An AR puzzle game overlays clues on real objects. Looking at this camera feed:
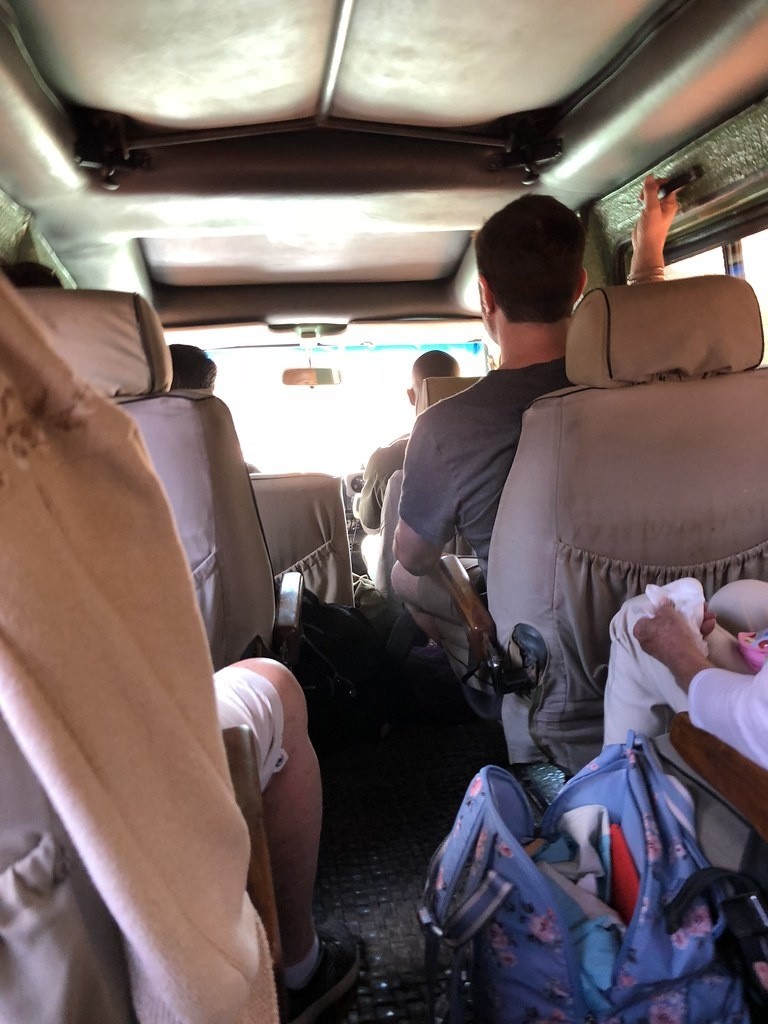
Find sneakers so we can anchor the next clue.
[274,920,360,1024]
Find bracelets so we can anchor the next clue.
[627,264,667,280]
[627,274,666,288]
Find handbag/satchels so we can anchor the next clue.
[301,588,395,736]
[417,730,768,1024]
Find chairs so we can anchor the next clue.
[433,275,768,838]
[13,286,304,684]
[647,711,768,888]
[247,472,356,608]
[361,376,484,605]
[221,722,288,1024]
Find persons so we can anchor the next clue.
[359,350,460,536]
[170,344,217,390]
[3,261,62,289]
[391,176,678,648]
[1,256,359,1024]
[601,577,768,769]
[282,369,318,385]
